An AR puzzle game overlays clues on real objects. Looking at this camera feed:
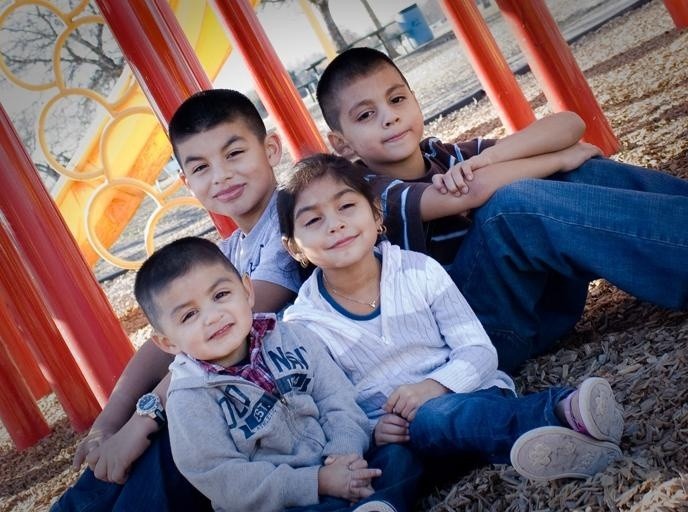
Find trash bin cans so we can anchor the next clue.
[397,3,434,46]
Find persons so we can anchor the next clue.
[277,153,626,481]
[50,90,311,511]
[134,236,419,512]
[316,47,686,375]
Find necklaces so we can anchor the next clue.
[319,273,383,310]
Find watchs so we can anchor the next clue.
[134,394,167,433]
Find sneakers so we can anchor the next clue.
[564,377,626,446]
[510,426,622,483]
